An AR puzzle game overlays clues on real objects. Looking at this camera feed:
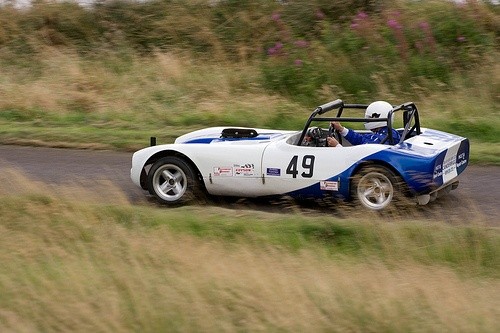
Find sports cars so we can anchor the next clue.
[130,99,470,217]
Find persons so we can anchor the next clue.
[326,101,401,148]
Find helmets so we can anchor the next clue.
[363,100,394,130]
